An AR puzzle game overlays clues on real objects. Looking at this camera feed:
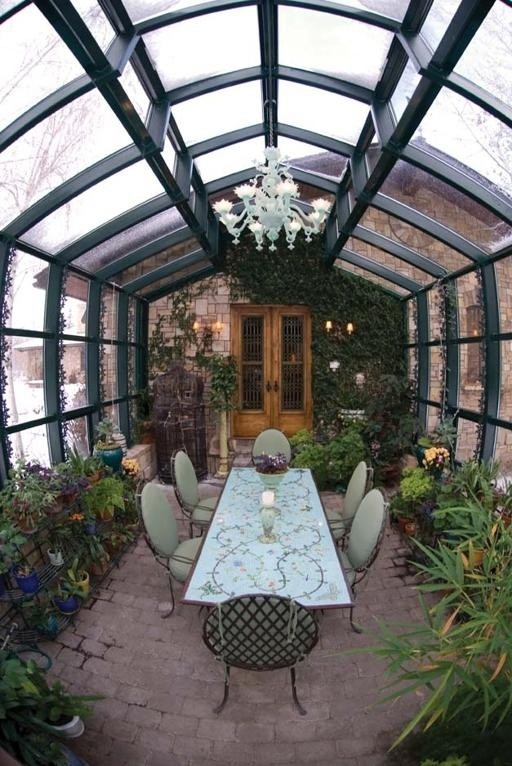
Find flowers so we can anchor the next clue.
[252,451,289,475]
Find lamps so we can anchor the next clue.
[211,98,332,253]
[192,321,223,353]
[325,321,354,372]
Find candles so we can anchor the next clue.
[262,488,274,507]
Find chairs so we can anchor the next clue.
[201,593,319,716]
[313,486,391,634]
[251,426,292,467]
[135,478,212,618]
[170,449,219,540]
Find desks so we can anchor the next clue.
[179,465,356,611]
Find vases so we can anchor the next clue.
[258,506,276,544]
[256,471,287,491]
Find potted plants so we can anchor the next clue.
[1,714,82,766]
[328,409,512,567]
[1,417,145,642]
[0,657,106,739]
